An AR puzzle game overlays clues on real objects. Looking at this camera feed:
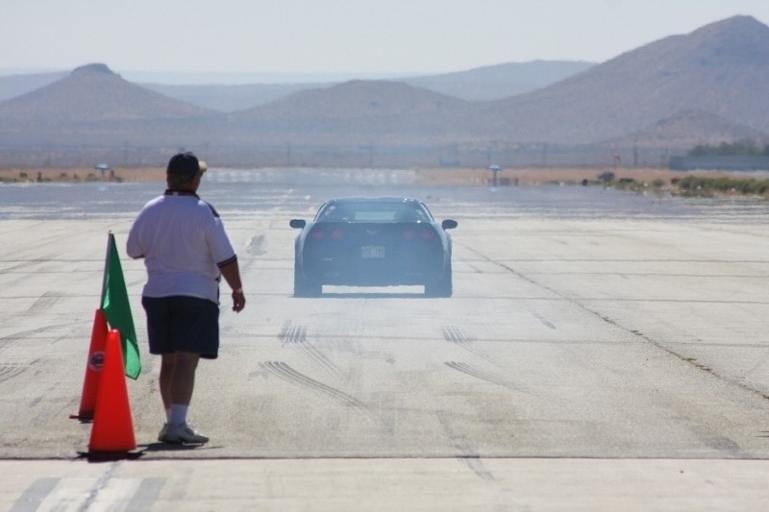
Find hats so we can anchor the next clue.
[168,154,206,176]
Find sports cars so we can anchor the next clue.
[290,196,457,298]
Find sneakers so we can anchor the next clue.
[158,423,168,441]
[164,421,208,442]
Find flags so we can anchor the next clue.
[100,233,141,380]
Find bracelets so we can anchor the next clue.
[235,288,242,294]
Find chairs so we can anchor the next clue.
[331,203,418,224]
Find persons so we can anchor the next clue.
[127,153,246,443]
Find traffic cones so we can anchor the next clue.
[78,331,147,458]
[71,308,108,420]
